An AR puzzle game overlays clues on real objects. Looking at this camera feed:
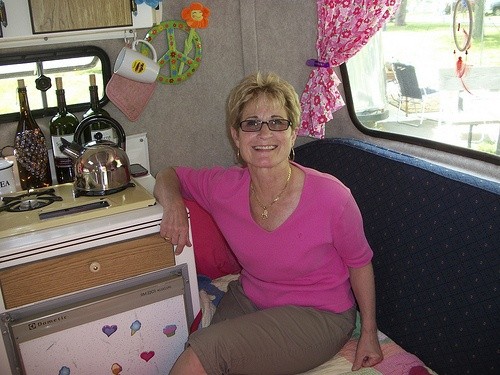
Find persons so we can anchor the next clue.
[152,70,384,375]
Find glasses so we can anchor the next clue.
[238,118,292,132]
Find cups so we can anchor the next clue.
[0,159,16,196]
[114,40,160,84]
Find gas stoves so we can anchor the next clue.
[0,175,156,240]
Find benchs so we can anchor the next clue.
[201,137,500,375]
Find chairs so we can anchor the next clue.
[381,60,441,126]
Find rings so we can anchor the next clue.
[164,236,172,240]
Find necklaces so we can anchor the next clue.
[251,166,291,217]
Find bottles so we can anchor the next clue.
[83,75,114,143]
[48,77,81,184]
[14,79,53,190]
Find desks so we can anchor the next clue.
[419,114,500,156]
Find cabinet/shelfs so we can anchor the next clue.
[0,231,201,375]
[28,0,134,34]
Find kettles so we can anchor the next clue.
[61,114,132,197]
